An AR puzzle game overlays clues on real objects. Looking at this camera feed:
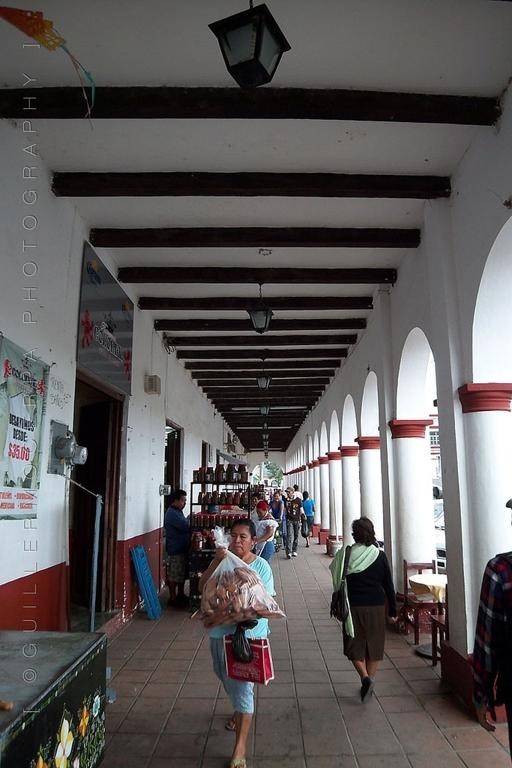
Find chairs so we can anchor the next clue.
[400,558,450,666]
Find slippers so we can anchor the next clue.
[225,713,237,731]
[230,757,247,767]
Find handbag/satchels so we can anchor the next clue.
[223,633,274,686]
[330,582,348,622]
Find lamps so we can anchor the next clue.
[247,282,274,458]
[207,1,292,91]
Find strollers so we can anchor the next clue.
[272,516,284,552]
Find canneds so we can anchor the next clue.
[191,463,249,552]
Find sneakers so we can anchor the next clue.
[287,551,297,558]
[168,594,189,608]
[360,678,374,703]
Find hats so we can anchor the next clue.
[257,501,267,508]
[285,486,295,492]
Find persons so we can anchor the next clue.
[196,518,277,768]
[164,489,195,609]
[331,516,400,703]
[249,484,316,563]
[471,552,511,760]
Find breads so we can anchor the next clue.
[200,567,286,627]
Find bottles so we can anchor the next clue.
[198,462,248,484]
[198,491,249,506]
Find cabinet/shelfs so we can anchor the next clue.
[188,480,249,600]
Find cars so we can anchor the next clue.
[433,481,447,576]
